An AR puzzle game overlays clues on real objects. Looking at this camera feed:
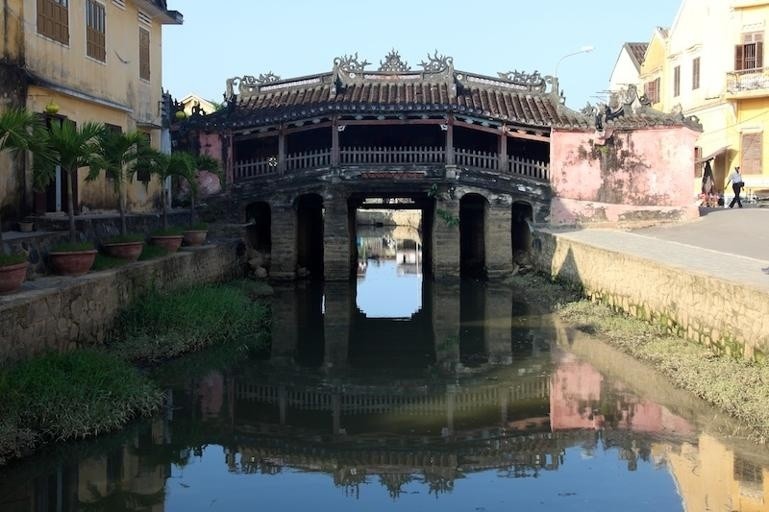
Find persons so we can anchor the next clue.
[723,165,746,208]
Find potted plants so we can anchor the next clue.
[125,149,229,255]
[1,107,148,295]
